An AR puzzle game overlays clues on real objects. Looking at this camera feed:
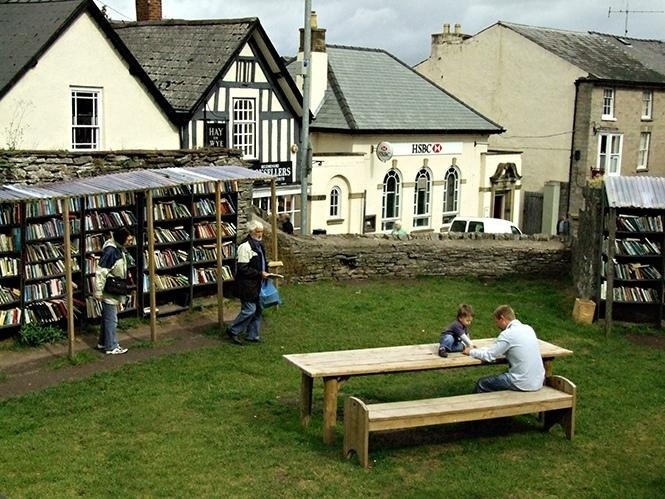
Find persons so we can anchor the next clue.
[389,220,407,235]
[556,214,567,236]
[280,214,295,235]
[93,227,134,356]
[227,220,270,343]
[438,303,479,359]
[462,304,548,393]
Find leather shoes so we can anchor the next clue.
[228,332,241,343]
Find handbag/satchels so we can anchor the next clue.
[105,276,127,295]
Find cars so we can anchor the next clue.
[449,215,526,235]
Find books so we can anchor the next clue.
[0,180,239,324]
[601,214,664,300]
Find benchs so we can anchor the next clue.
[343,375,577,468]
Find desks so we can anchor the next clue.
[282,338,573,444]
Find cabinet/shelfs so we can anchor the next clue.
[1,165,279,356]
[596,175,665,337]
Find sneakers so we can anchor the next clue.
[440,352,448,356]
[98,344,105,348]
[106,344,128,354]
